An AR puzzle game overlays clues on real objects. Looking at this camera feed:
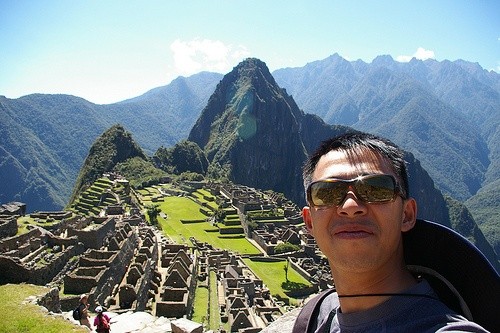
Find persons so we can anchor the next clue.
[79,294,91,331]
[259,133,493,333]
[94,306,111,333]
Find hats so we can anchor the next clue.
[403,218,500,333]
[79,294,89,301]
[95,306,103,312]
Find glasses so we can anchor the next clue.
[306,174,407,211]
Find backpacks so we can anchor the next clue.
[96,315,111,333]
[73,304,86,320]
